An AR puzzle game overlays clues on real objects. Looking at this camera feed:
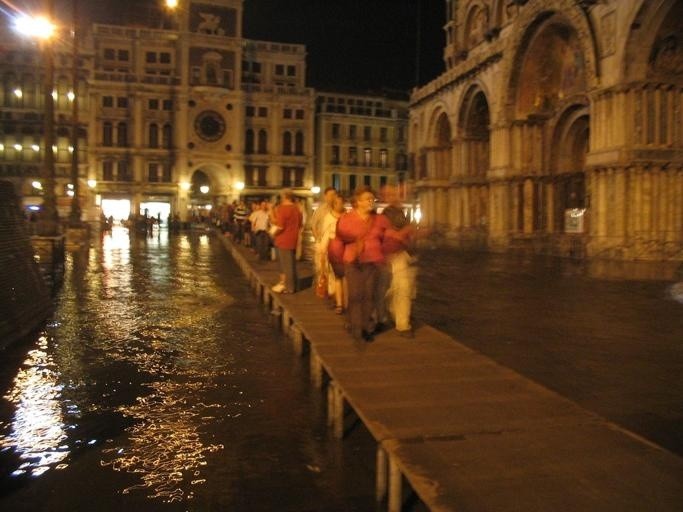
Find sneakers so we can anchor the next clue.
[273,281,296,293]
[393,329,415,338]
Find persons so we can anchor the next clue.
[190,183,423,348]
